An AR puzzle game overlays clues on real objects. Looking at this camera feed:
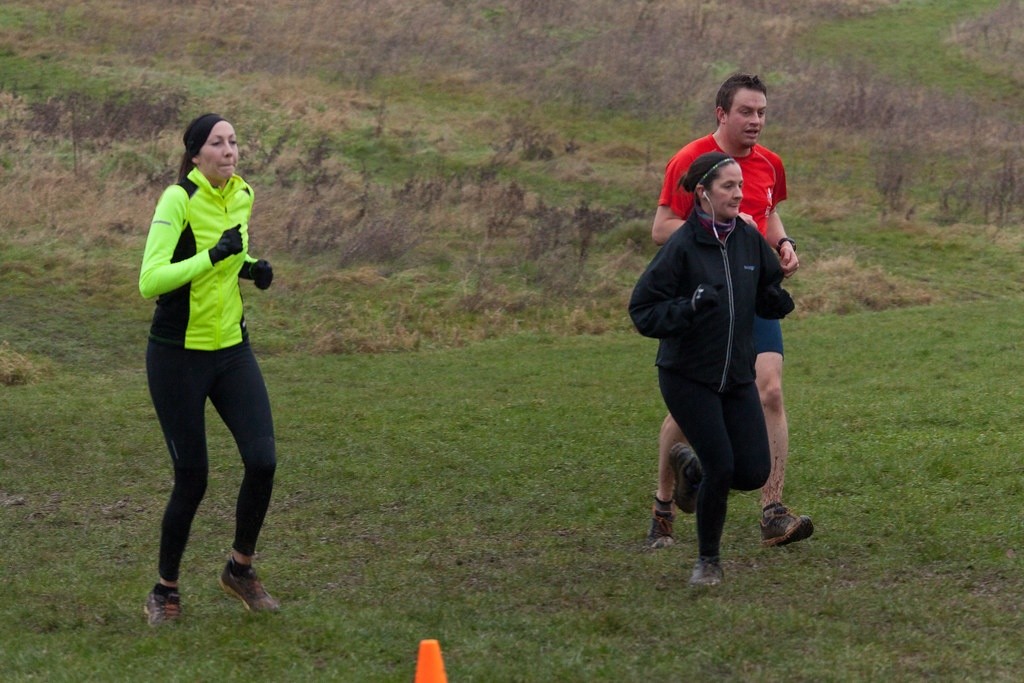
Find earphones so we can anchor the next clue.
[703,192,711,203]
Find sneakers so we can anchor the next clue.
[689,558,722,587]
[646,506,673,539]
[669,442,697,515]
[218,561,279,616]
[143,593,180,628]
[760,509,813,548]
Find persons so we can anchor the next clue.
[650,74,813,548]
[628,153,796,586]
[140,113,279,618]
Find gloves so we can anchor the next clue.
[692,284,719,318]
[208,224,244,265]
[778,289,796,316]
[252,258,272,290]
